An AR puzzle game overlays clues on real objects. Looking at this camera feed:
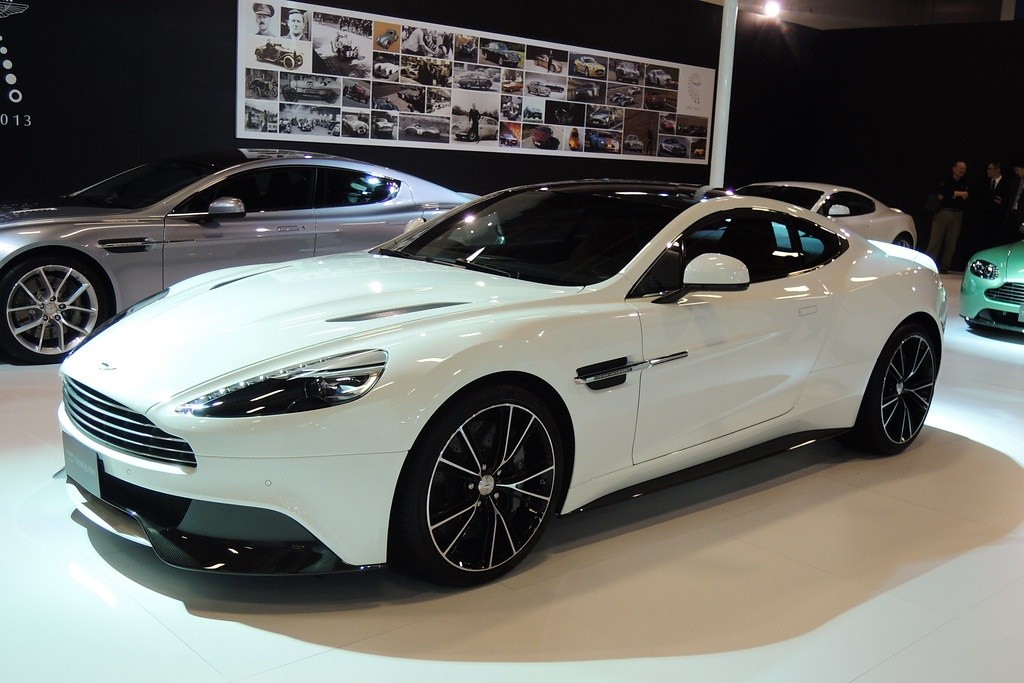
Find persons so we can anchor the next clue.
[547,50,553,73]
[645,124,654,156]
[457,34,476,54]
[926,162,969,273]
[339,16,372,37]
[286,10,308,41]
[253,3,275,36]
[469,102,482,143]
[247,109,278,132]
[401,25,453,59]
[983,159,1024,249]
[508,102,518,120]
[400,57,452,85]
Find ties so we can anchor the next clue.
[992,180,995,189]
[1011,180,1024,211]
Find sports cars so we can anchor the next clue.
[405,123,441,138]
[59,181,932,585]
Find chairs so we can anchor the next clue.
[711,216,779,279]
[544,198,641,264]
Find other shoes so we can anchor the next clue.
[940,268,947,274]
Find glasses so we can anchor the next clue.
[987,168,997,170]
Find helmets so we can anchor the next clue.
[355,82,357,85]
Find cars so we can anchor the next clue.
[501,79,564,149]
[590,131,620,152]
[647,91,667,106]
[0,148,507,372]
[958,240,1024,334]
[343,114,369,137]
[376,29,399,49]
[253,41,303,70]
[458,71,492,90]
[647,69,671,87]
[589,108,617,129]
[613,86,642,107]
[660,118,707,159]
[616,62,641,85]
[481,41,521,66]
[625,134,644,152]
[690,183,918,258]
[373,117,394,134]
[535,54,564,72]
[574,56,606,78]
[569,128,581,151]
[452,116,498,141]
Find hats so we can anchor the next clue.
[252,2,275,17]
